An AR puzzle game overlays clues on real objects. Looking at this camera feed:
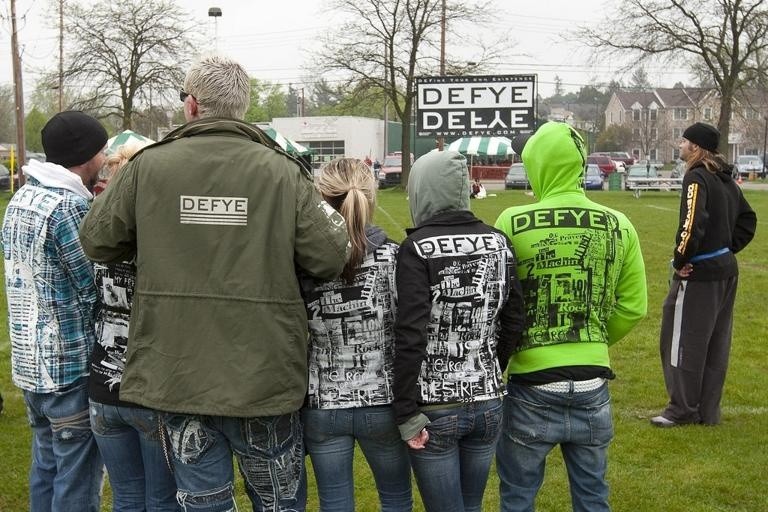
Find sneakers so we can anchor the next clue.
[649,414,678,427]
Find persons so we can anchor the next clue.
[731,162,740,182]
[493,122,647,512]
[80,58,351,512]
[87,147,181,511]
[1,110,104,512]
[651,123,757,426]
[295,159,412,512]
[394,150,525,512]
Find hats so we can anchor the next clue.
[683,121,722,155]
[40,110,109,168]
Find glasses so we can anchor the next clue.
[179,88,196,104]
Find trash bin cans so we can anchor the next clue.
[608,173,621,191]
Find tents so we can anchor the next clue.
[106,129,157,155]
[451,136,517,179]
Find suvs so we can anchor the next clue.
[377,151,414,189]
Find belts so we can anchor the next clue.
[528,375,608,395]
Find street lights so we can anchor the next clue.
[166,108,174,132]
[561,100,570,119]
[208,7,222,57]
[631,72,649,155]
[589,85,598,153]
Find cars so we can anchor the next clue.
[0,162,30,192]
[503,162,532,189]
[580,151,768,191]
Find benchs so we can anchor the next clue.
[625,176,682,198]
